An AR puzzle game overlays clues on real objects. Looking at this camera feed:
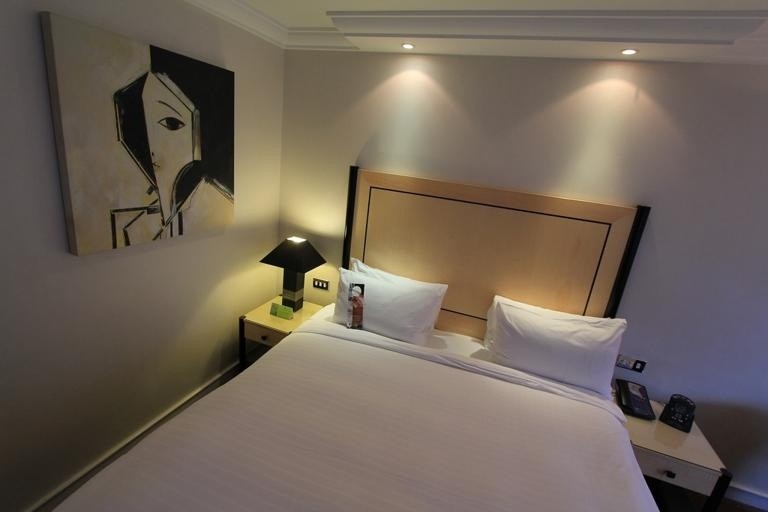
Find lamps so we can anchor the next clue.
[259,235,327,312]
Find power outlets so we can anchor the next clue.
[615,353,647,374]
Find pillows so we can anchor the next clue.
[347,258,439,285]
[333,268,451,346]
[484,296,629,399]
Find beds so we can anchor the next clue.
[59,162,661,512]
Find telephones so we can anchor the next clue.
[615,379,655,420]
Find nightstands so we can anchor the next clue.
[613,390,733,512]
[241,295,324,373]
